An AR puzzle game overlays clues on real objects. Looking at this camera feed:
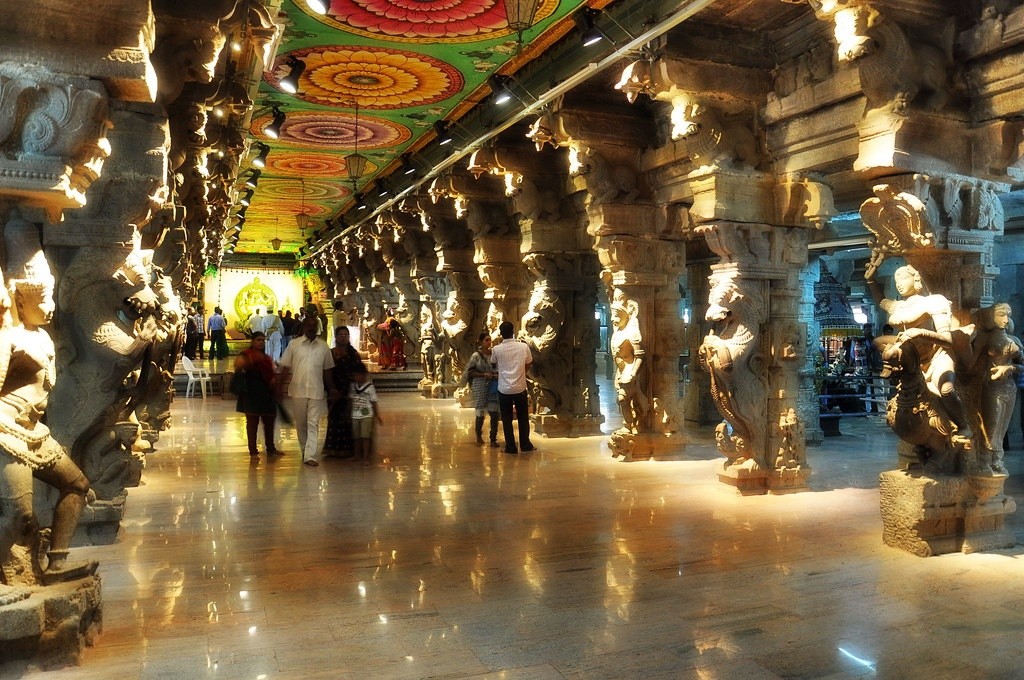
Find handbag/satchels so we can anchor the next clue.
[492,379,500,393]
[229,351,251,393]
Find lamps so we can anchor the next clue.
[433,118,476,145]
[306,0,330,14]
[271,221,282,250]
[227,168,261,253]
[251,141,271,167]
[488,73,538,107]
[573,5,656,64]
[374,178,388,197]
[302,220,335,260]
[400,152,433,174]
[279,57,306,94]
[354,193,367,210]
[503,0,539,45]
[265,105,287,138]
[342,104,368,190]
[296,181,311,236]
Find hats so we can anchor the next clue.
[864,324,872,329]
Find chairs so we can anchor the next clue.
[182,355,212,399]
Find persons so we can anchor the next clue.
[185,306,206,360]
[485,312,501,350]
[883,264,1024,474]
[863,323,893,384]
[0,278,97,578]
[611,304,649,434]
[207,309,228,360]
[491,322,537,453]
[377,311,408,369]
[418,311,439,383]
[467,333,501,447]
[247,305,324,366]
[236,317,384,466]
